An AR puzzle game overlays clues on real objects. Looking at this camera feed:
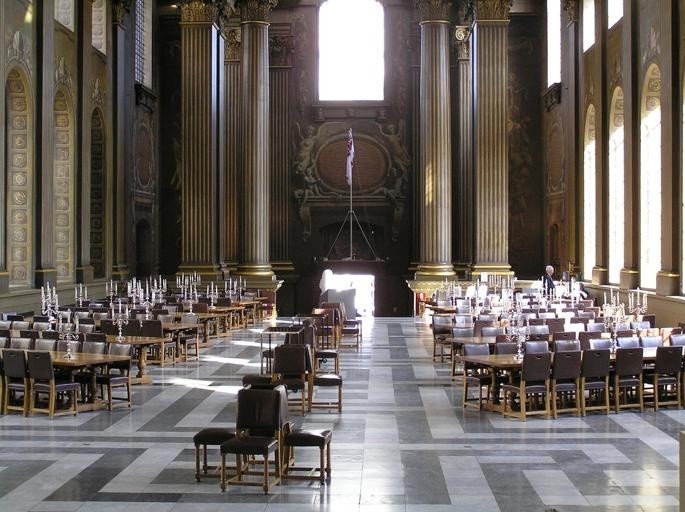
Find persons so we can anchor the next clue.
[370,170,403,212]
[292,119,319,174]
[541,263,559,294]
[375,119,413,170]
[299,167,321,209]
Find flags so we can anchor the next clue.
[344,126,358,189]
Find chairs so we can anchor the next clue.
[416,285,685,424]
